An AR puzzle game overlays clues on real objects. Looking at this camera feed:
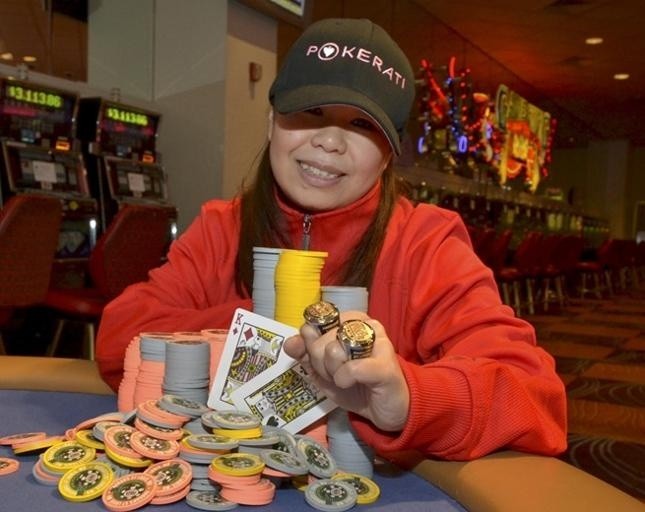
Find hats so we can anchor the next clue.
[268,17,416,158]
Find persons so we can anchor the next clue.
[94,17,568,472]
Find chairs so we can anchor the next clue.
[0,191,181,360]
[471,227,645,319]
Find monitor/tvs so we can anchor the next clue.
[2,138,91,199]
[100,155,170,204]
[237,0,314,32]
[43,0,89,25]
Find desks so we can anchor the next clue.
[1,353,645,512]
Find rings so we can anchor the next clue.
[303,300,339,335]
[336,319,375,360]
